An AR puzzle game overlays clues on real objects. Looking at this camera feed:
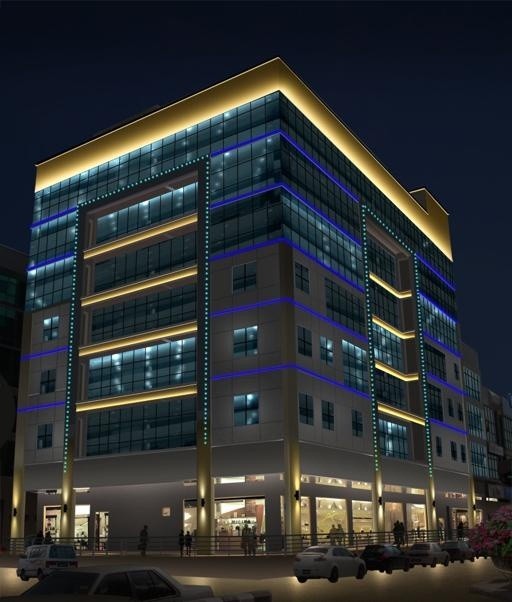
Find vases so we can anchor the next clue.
[489,556,512,570]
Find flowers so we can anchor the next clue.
[471,502,512,574]
